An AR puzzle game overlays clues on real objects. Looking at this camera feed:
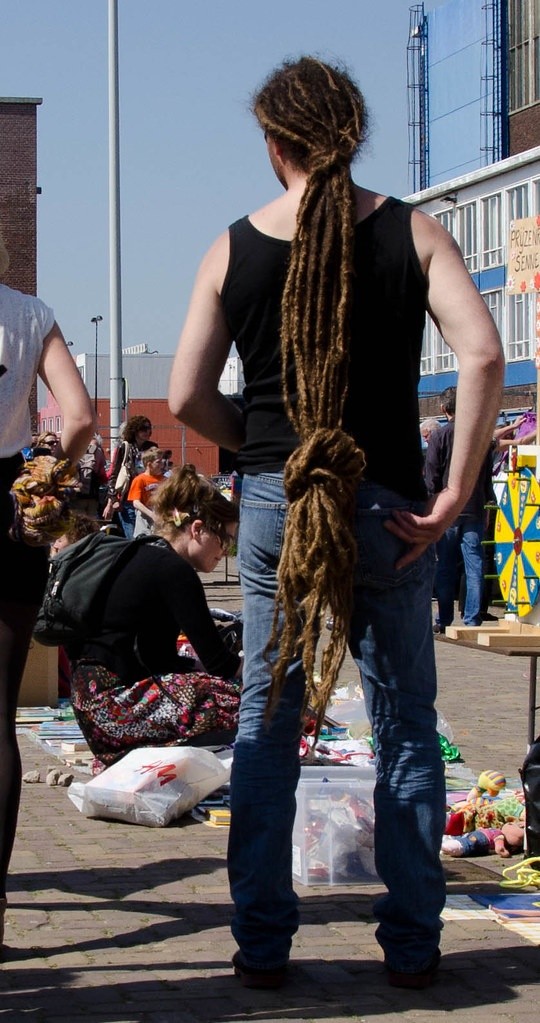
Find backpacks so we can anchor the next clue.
[34,528,173,647]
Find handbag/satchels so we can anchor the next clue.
[115,441,130,494]
[81,747,232,828]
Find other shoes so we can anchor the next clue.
[480,612,498,621]
[431,623,445,634]
[231,950,287,988]
[386,946,440,988]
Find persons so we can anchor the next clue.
[0,241,98,954]
[22,415,242,766]
[420,387,537,634]
[169,57,506,988]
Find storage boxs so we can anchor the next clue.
[291,766,385,886]
[17,634,60,707]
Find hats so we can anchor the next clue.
[142,446,173,466]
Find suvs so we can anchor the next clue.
[209,476,232,489]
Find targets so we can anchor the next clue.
[492,466,539,619]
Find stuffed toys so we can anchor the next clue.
[441,771,525,857]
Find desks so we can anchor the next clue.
[438,618,540,859]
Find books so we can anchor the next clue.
[203,806,231,826]
[15,707,89,751]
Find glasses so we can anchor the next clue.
[42,440,59,446]
[185,517,236,551]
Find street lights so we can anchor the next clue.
[90,316,103,413]
